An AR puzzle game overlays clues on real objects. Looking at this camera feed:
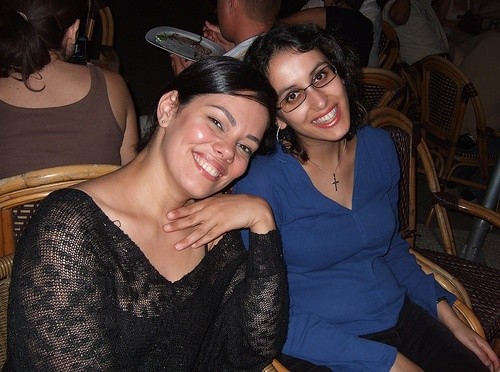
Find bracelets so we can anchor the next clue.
[434,296,447,304]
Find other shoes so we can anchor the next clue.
[460,190,478,204]
[444,187,460,199]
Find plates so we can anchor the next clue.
[145,25,226,63]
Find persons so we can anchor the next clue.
[2,56,289,372]
[233,24,500,372]
[0,0,139,180]
[169,0,456,87]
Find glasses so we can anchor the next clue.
[277,60,338,112]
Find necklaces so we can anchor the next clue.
[309,143,343,191]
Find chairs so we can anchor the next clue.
[0,0,500,372]
